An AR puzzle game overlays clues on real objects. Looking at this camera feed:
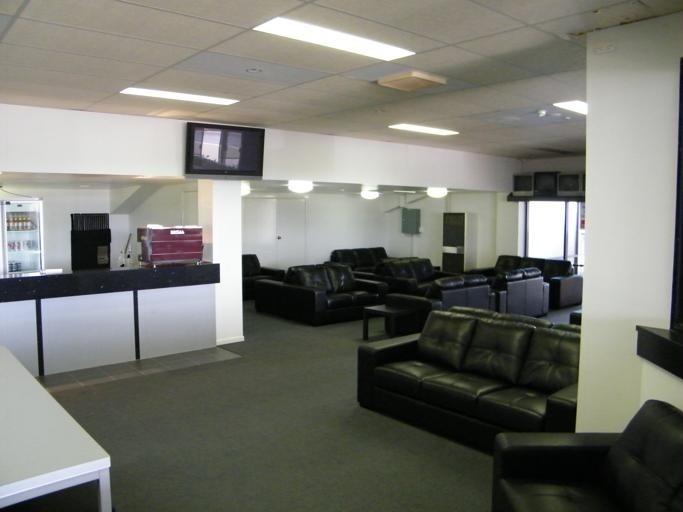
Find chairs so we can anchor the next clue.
[492,399,683,511]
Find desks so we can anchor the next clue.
[1,346,112,512]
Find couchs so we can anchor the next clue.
[255,263,390,328]
[463,254,582,310]
[361,266,552,344]
[323,248,420,281]
[243,253,287,305]
[385,256,462,296]
[353,304,583,457]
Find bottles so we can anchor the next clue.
[7,215,32,231]
[7,240,37,252]
[7,259,22,272]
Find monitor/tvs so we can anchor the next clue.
[185,121,265,178]
[511,172,534,197]
[558,172,584,197]
[534,171,558,197]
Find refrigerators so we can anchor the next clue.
[0,198,43,274]
